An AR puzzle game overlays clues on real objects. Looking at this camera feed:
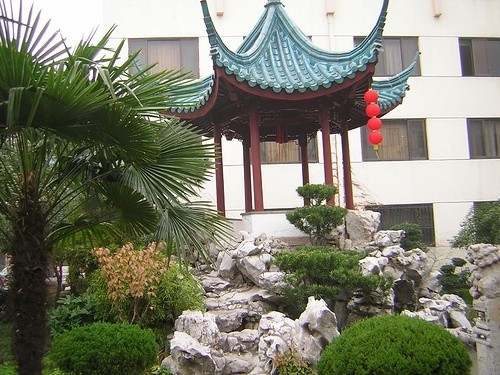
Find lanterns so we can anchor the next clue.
[364,87,383,159]
